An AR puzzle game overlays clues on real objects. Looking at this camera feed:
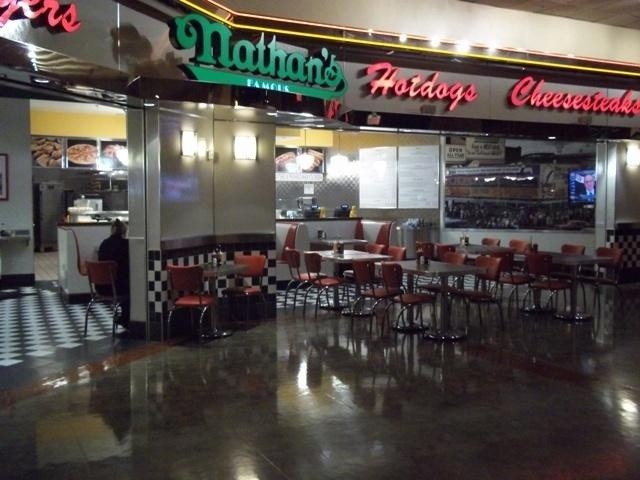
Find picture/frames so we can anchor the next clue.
[0,153,10,201]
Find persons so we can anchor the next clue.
[95,217,130,329]
[93,337,136,443]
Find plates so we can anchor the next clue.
[66,144,97,165]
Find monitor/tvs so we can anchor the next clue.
[568,167,596,204]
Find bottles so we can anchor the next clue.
[333,239,344,255]
[416,245,425,265]
[213,245,224,267]
[349,205,359,217]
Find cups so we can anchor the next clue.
[320,207,326,218]
